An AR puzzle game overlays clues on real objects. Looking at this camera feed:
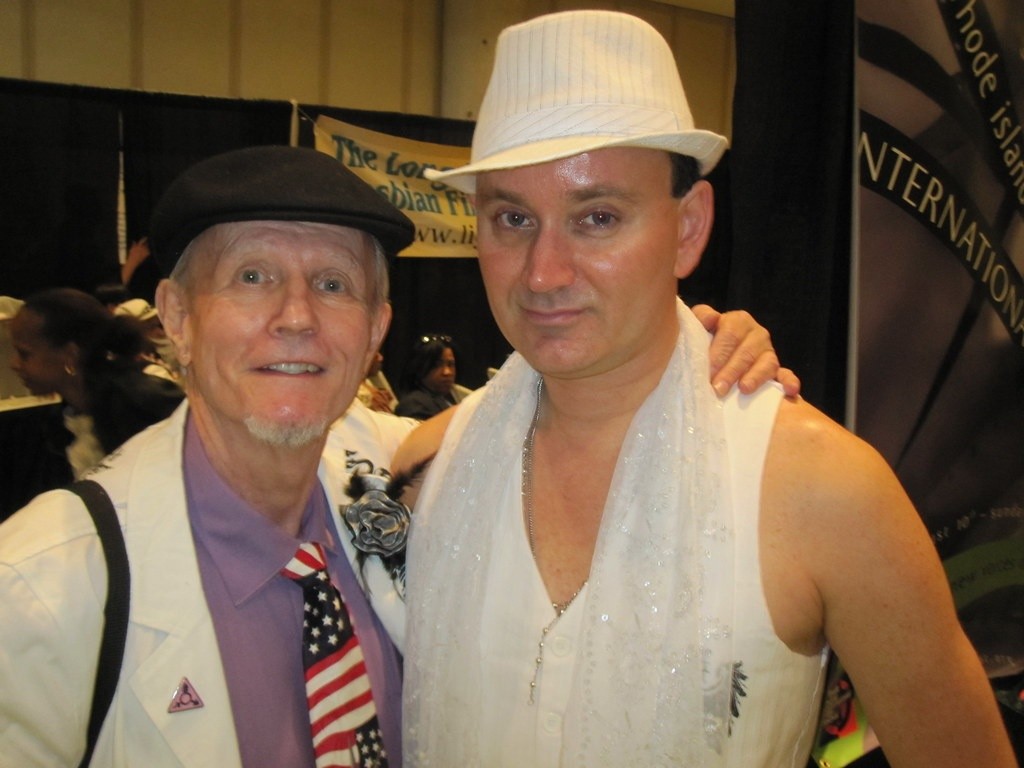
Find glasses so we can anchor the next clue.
[421,335,453,343]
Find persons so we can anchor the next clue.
[0,145,799,768]
[356,352,398,414]
[390,10,1019,768]
[394,332,474,422]
[7,236,186,513]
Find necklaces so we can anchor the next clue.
[521,377,586,705]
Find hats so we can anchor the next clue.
[147,144,415,278]
[422,9,729,194]
